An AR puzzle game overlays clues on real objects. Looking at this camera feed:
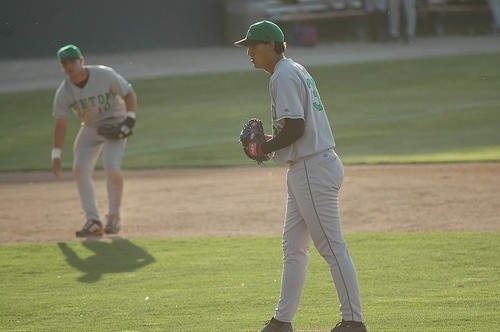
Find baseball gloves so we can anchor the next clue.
[239,117,274,163]
[97,125,134,141]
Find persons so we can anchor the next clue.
[52,45,137,236]
[234,20,367,332]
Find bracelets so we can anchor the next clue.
[52,149,61,158]
[127,112,136,119]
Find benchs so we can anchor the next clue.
[415,5,492,14]
[268,8,362,22]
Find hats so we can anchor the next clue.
[57,44,82,63]
[235,20,284,46]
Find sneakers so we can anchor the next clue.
[76,219,102,237]
[331,322,366,332]
[105,218,121,233]
[261,317,293,332]
